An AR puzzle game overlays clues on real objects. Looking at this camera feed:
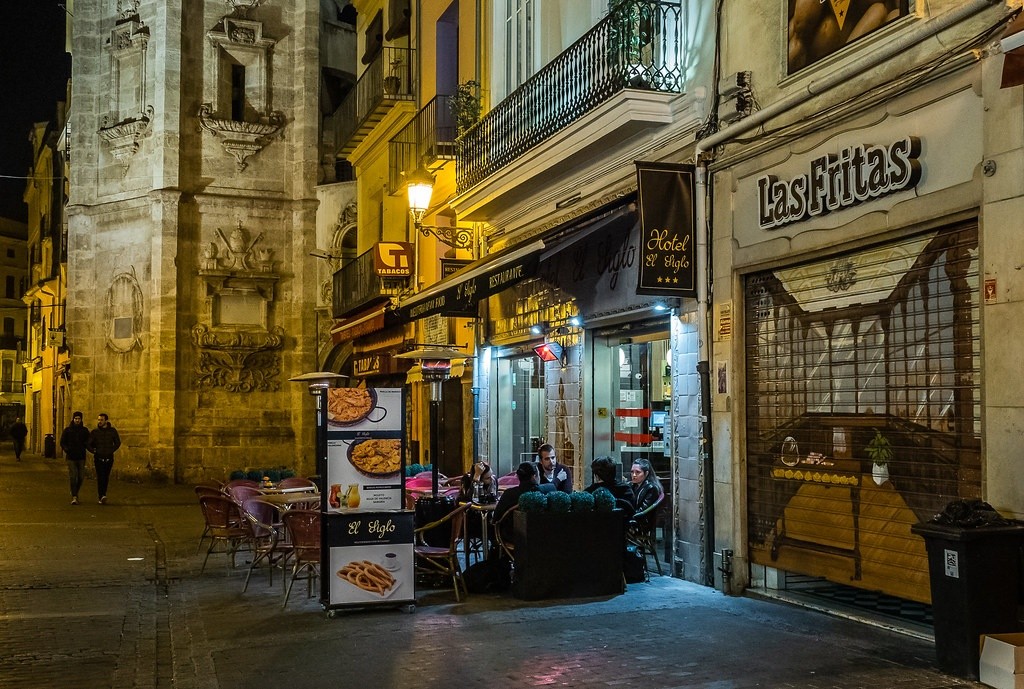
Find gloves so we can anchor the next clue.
[557,468,567,481]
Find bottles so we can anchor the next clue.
[258,481,273,488]
[473,482,484,499]
[329,483,361,509]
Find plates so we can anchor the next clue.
[380,560,401,572]
[335,563,402,599]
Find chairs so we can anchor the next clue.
[613,481,665,580]
[194,476,322,607]
[412,501,472,604]
[490,504,519,592]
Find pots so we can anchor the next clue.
[342,439,401,478]
[327,389,387,427]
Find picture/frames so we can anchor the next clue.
[775,1,925,89]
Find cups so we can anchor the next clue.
[383,552,399,568]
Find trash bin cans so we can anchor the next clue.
[44,434,56,458]
[911,520,1024,681]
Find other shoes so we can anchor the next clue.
[98,496,107,504]
[70,497,79,504]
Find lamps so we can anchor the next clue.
[403,160,472,251]
[383,52,404,96]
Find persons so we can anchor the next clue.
[60,412,90,505]
[487,461,557,584]
[457,461,499,538]
[627,458,660,534]
[10,417,28,462]
[536,444,572,495]
[86,413,121,504]
[583,456,636,523]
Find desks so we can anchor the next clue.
[254,486,315,536]
[256,489,322,583]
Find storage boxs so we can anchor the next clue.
[979,634,1024,689]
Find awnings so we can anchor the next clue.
[331,300,390,346]
[400,199,621,324]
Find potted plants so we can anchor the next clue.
[513,486,626,602]
[447,79,486,159]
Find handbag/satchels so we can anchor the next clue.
[94,455,114,465]
